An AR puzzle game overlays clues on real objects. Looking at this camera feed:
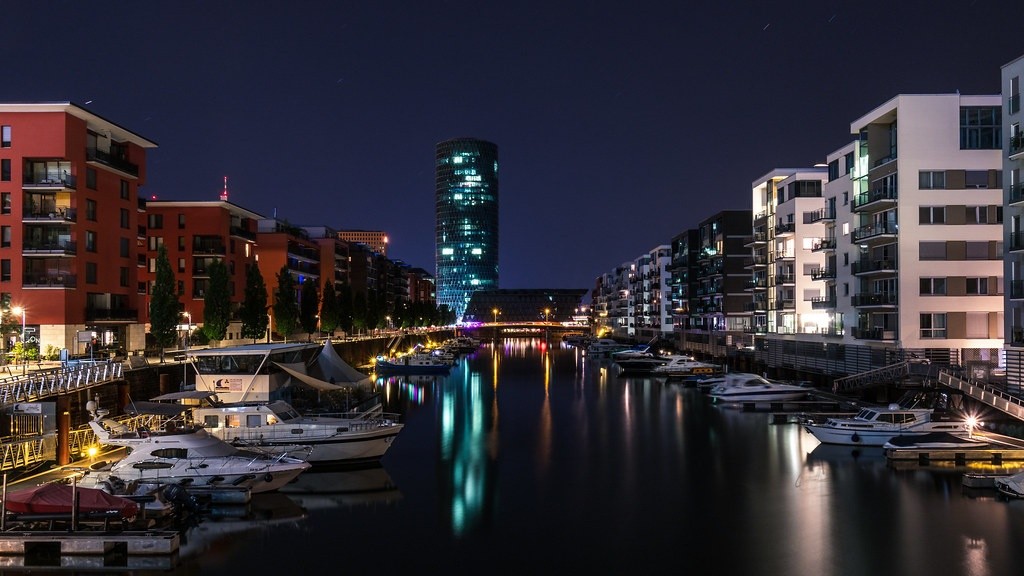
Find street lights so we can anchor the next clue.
[182,311,192,346]
[492,308,499,322]
[545,308,550,321]
[10,304,27,349]
[315,315,320,337]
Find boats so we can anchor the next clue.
[67,393,314,495]
[0,473,180,555]
[563,334,634,353]
[798,390,969,447]
[149,391,404,469]
[375,336,480,375]
[612,335,815,403]
[38,448,201,531]
[883,432,991,451]
[1,481,137,513]
